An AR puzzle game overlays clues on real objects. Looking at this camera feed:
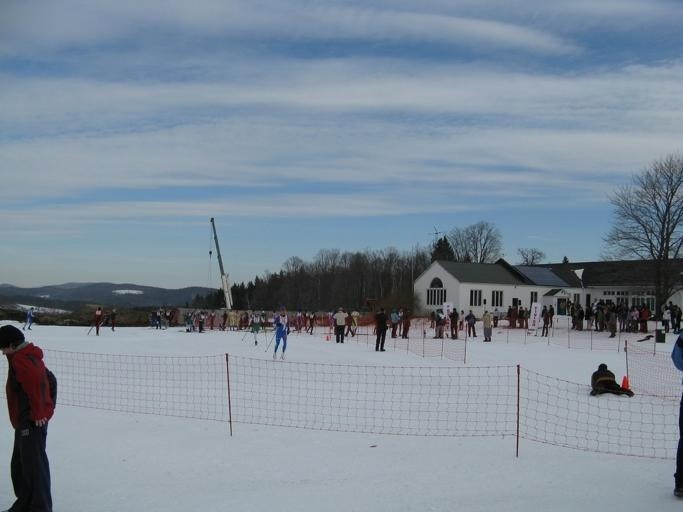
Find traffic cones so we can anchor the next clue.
[325,336,329,341]
[620,376,627,388]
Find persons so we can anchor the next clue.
[506,304,533,329]
[95,307,102,336]
[374,306,410,352]
[155,306,173,330]
[220,309,266,334]
[493,307,499,328]
[483,310,492,342]
[590,363,635,397]
[23,307,35,331]
[0,325,57,512]
[185,309,216,333]
[671,331,683,497]
[541,304,554,337]
[111,308,117,331]
[559,299,651,338]
[273,306,289,360]
[661,300,682,334]
[332,306,359,343]
[429,305,478,339]
[293,307,317,335]
